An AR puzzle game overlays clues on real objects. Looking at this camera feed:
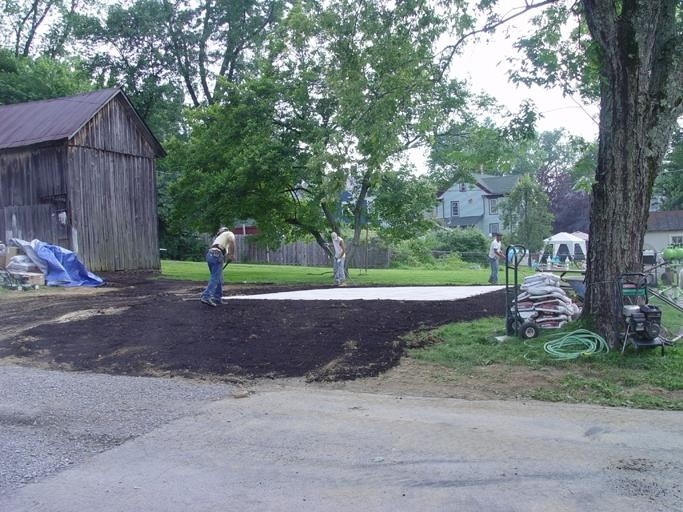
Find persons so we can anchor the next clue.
[488,232,506,284]
[323,232,346,285]
[199,226,236,307]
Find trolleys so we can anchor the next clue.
[501,243,556,338]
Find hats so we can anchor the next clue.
[217,227,228,235]
[331,232,337,240]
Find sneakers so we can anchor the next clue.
[201,297,228,307]
